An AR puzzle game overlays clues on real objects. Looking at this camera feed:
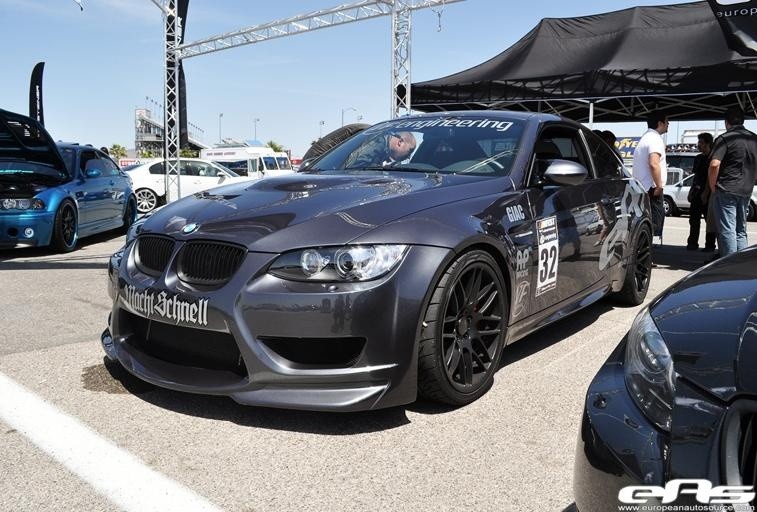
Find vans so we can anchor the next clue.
[201,147,295,186]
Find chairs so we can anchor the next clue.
[535,141,562,157]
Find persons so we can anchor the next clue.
[686,132,717,252]
[301,124,416,168]
[703,105,757,264]
[634,110,669,268]
[594,128,624,161]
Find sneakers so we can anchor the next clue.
[703,256,721,264]
[686,244,714,251]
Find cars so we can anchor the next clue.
[100,110,654,414]
[573,237,756,509]
[663,170,756,220]
[0,111,139,254]
[121,158,248,213]
